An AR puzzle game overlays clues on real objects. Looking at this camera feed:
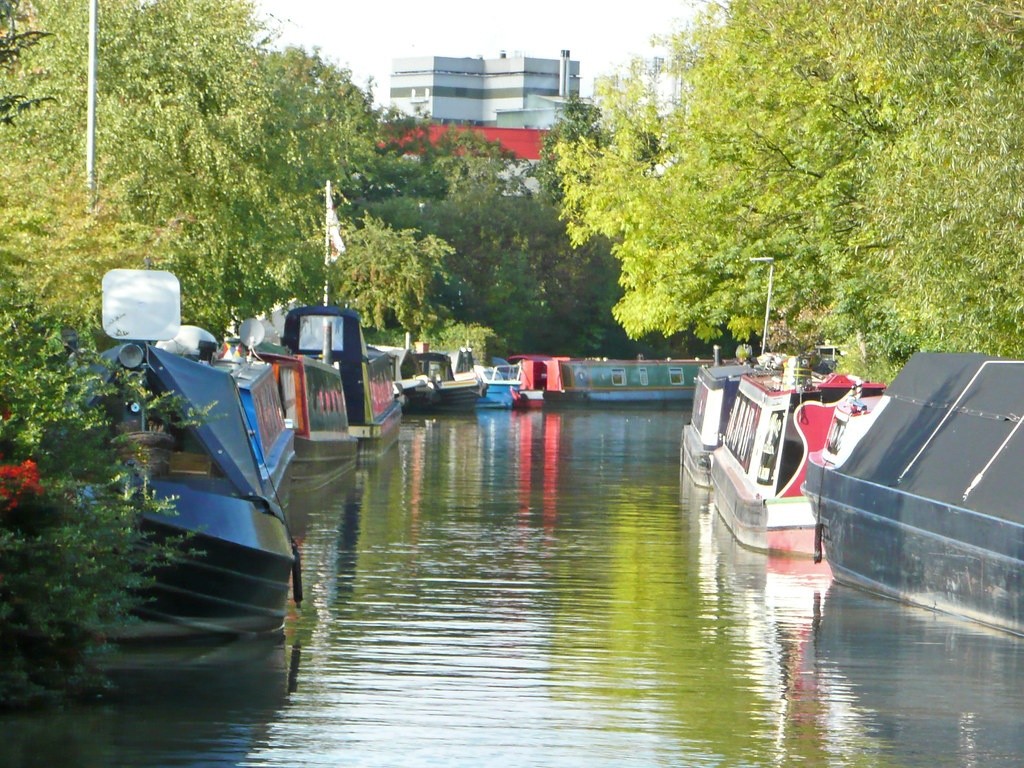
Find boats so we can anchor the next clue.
[678,256,888,557]
[0,180,523,639]
[802,354,1024,638]
[508,356,758,402]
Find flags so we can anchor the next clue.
[326,193,346,263]
